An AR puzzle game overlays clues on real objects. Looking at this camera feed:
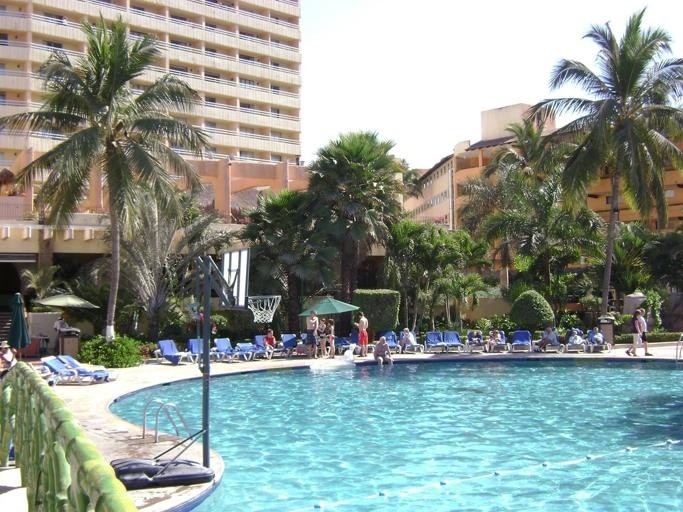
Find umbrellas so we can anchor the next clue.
[7,292,31,360]
[35,291,100,324]
[298,296,360,317]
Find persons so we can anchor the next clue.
[318,318,328,358]
[261,327,276,358]
[533,326,556,352]
[304,310,319,360]
[353,311,369,357]
[569,330,586,344]
[631,308,653,356]
[0,339,17,369]
[488,330,500,345]
[471,330,482,343]
[373,335,394,366]
[326,318,335,360]
[587,327,605,345]
[625,309,643,356]
[399,328,415,346]
[52,316,65,355]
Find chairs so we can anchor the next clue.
[39,356,94,384]
[57,354,110,382]
[154,330,607,365]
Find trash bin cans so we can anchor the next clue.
[598,317,615,345]
[58,328,80,355]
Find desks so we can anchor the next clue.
[24,336,49,357]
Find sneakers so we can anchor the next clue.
[625,350,653,357]
